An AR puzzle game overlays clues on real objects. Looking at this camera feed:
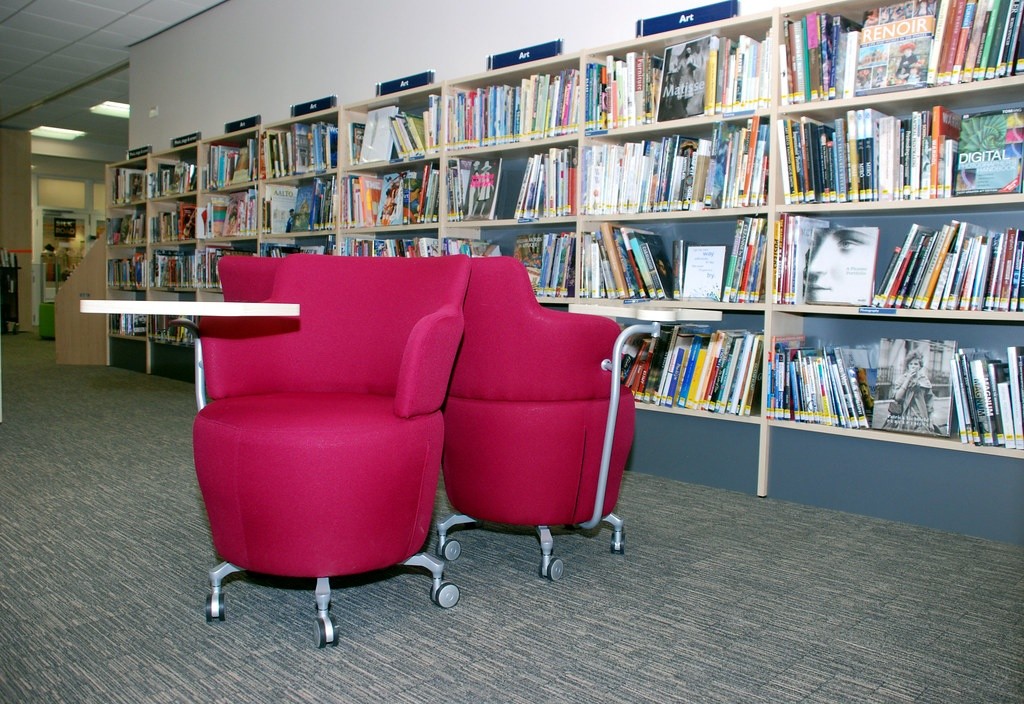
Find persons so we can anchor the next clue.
[882,352,935,433]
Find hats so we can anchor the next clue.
[481,161,492,171]
[473,161,480,171]
[899,43,916,53]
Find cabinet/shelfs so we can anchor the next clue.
[104,0,1024,547]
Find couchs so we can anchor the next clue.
[443,253,638,586]
[190,248,476,655]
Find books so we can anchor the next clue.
[0,247,16,267]
[109,0,1024,453]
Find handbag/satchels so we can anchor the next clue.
[888,402,902,416]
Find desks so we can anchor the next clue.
[557,299,728,327]
[76,294,303,322]
